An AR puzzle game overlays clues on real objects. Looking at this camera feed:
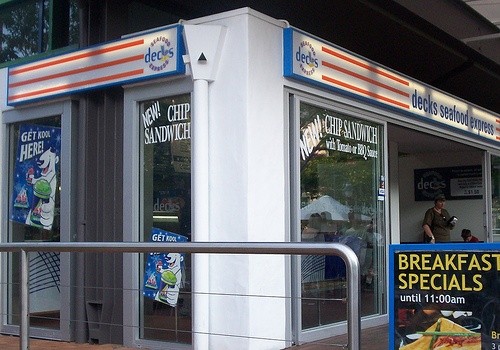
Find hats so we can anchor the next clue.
[434,194,446,202]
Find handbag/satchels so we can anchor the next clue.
[424,231,432,243]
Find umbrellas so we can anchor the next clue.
[301,195,373,220]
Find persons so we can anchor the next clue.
[422,190,459,244]
[461,229,479,243]
[300,210,375,305]
